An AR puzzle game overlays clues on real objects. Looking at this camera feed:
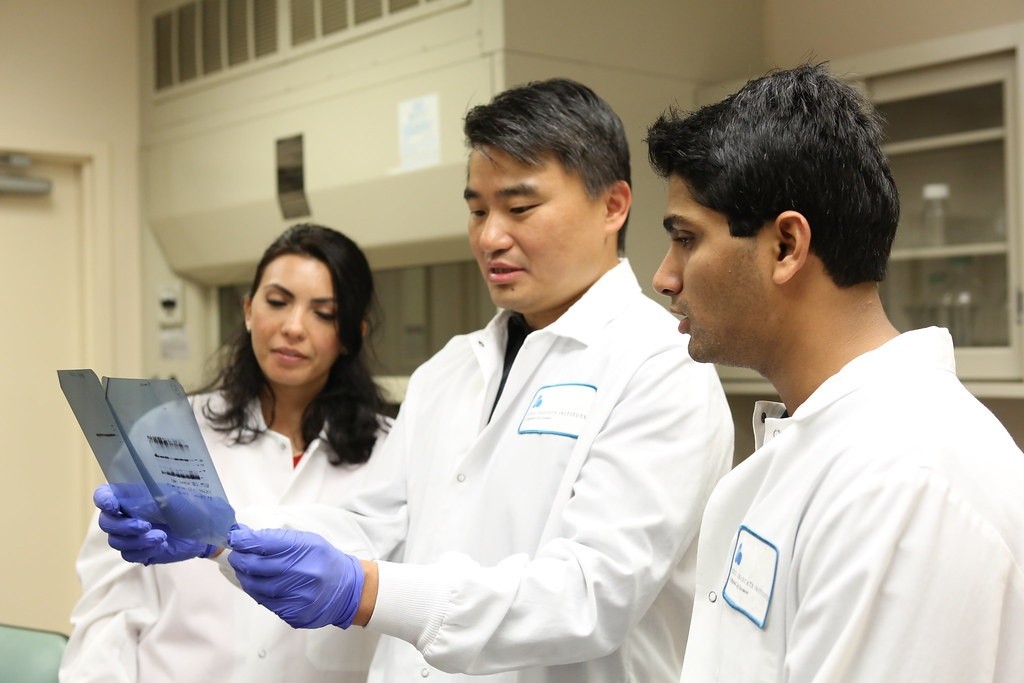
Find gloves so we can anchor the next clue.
[226,523,364,630]
[93,483,235,567]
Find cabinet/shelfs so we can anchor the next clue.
[852,19,1024,383]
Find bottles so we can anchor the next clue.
[926,266,975,345]
[921,183,959,243]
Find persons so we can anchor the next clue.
[646,64,1024,683]
[92,79,735,683]
[57,223,397,683]
